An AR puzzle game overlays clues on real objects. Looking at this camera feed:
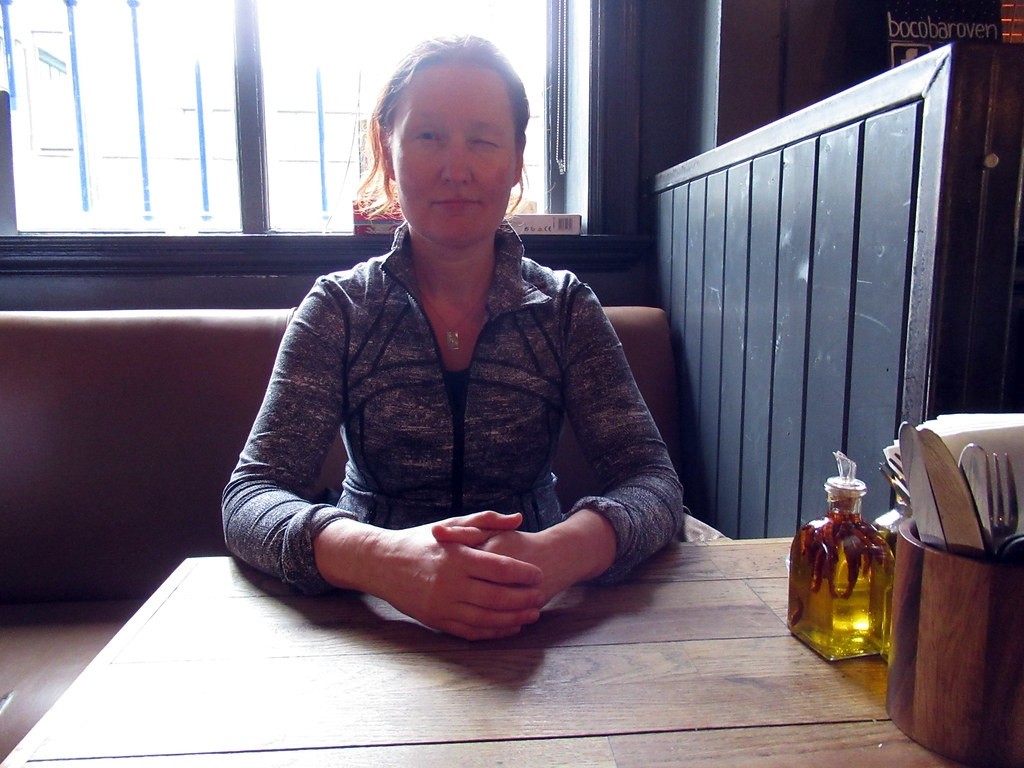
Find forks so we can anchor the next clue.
[986,452,1019,535]
[879,453,911,517]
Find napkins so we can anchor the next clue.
[897,411,1024,535]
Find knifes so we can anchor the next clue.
[897,421,992,556]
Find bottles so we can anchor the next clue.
[788,452,894,661]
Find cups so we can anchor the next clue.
[886,520,1024,768]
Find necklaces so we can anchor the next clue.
[422,292,479,350]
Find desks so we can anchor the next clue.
[1,536,976,768]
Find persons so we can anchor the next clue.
[221,33,683,637]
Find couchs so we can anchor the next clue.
[1,307,734,768]
